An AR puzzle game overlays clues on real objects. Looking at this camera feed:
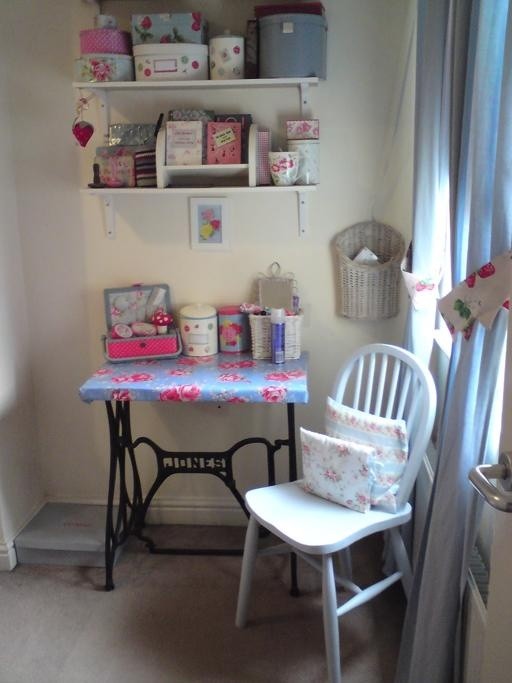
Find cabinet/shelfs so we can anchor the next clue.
[73,76,319,238]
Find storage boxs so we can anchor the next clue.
[15,503,127,568]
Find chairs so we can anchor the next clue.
[234,342,436,683]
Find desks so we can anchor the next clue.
[78,351,308,599]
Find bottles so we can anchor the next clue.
[271,306,285,364]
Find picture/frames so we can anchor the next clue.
[191,195,227,252]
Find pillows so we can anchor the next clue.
[301,395,408,514]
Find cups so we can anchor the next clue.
[179,304,219,356]
[268,150,309,186]
[209,29,245,79]
